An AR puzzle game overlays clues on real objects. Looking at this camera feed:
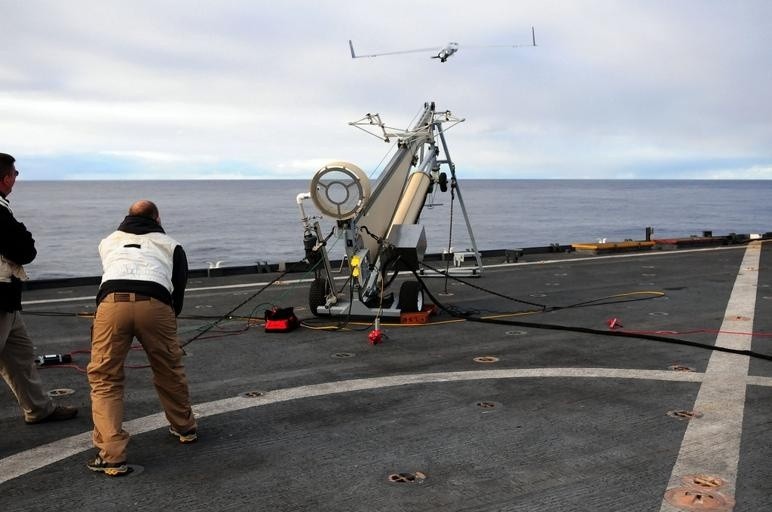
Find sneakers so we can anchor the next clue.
[84,450,129,477]
[23,404,80,424]
[167,422,199,443]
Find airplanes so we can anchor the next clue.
[349,26,535,63]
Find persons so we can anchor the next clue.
[0,152,78,425]
[86,199,198,475]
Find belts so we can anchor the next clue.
[113,292,152,303]
